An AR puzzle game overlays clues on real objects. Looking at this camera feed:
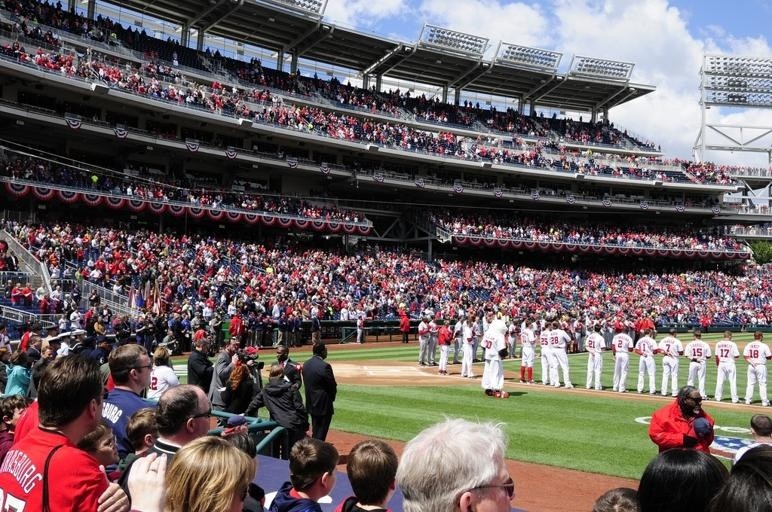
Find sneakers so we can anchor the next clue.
[419,356,480,379]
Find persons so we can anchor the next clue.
[585,323,605,390]
[0,0,772,333]
[715,330,744,403]
[658,328,684,398]
[591,386,772,511]
[634,329,658,395]
[2,313,515,512]
[684,331,711,400]
[743,331,770,406]
[612,325,633,393]
[519,321,576,388]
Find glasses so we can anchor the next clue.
[689,397,702,402]
[128,363,152,372]
[182,408,211,423]
[457,477,514,507]
[240,485,247,501]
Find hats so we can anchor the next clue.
[227,416,246,426]
[245,347,257,355]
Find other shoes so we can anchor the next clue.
[704,395,769,407]
[519,378,573,389]
[587,385,677,398]
